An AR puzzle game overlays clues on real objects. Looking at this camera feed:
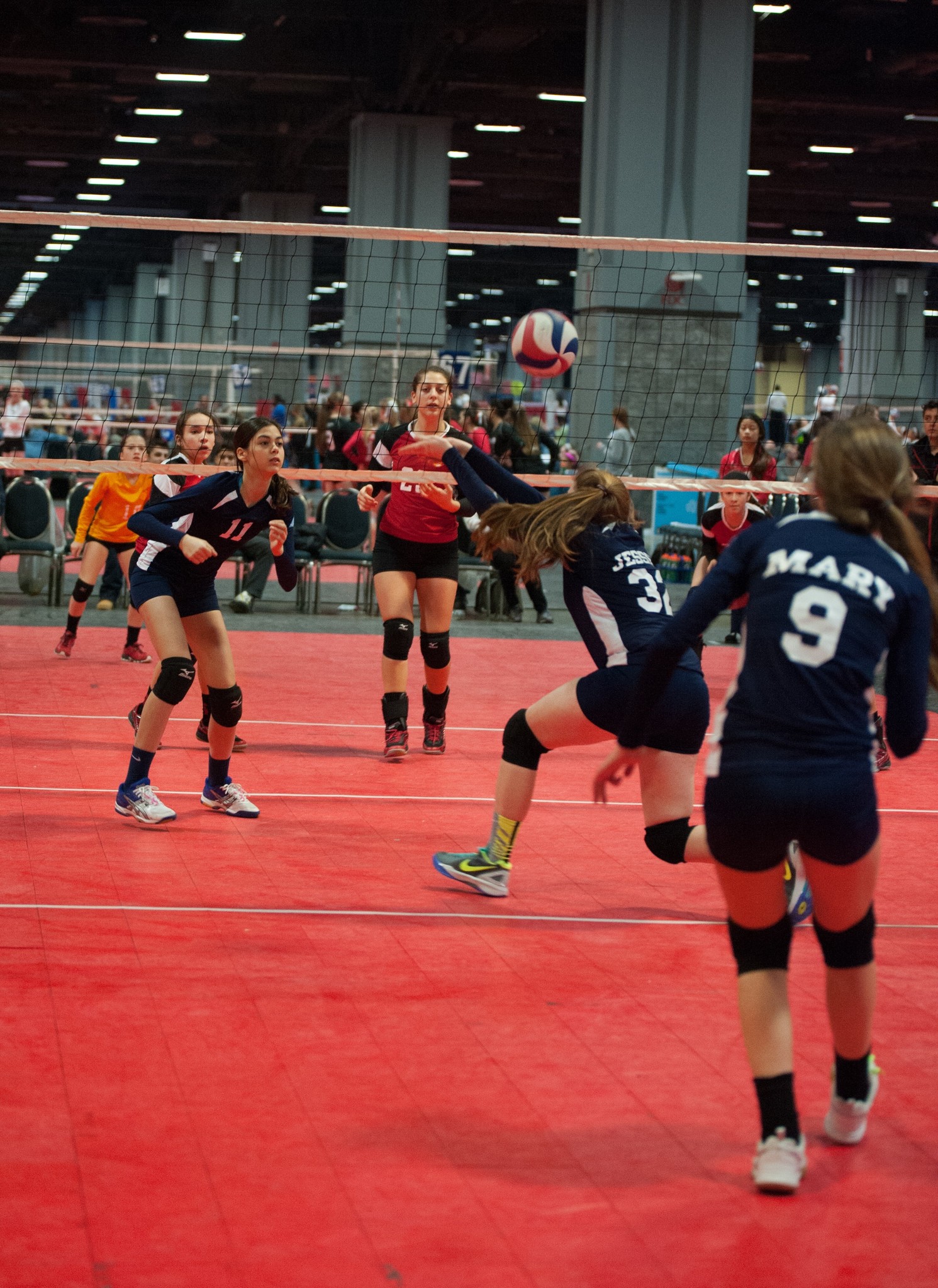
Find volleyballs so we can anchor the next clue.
[510,307,577,381]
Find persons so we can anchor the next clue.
[357,364,479,761]
[588,417,938,1193]
[397,427,816,928]
[127,411,248,753]
[114,421,306,826]
[0,382,938,671]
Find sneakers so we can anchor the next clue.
[114,777,176,824]
[53,631,76,656]
[120,641,153,664]
[195,719,247,750]
[383,718,410,757]
[433,848,511,898]
[422,715,447,754]
[127,703,162,749]
[751,1127,807,1191]
[199,776,260,818]
[824,1056,880,1145]
[783,841,813,924]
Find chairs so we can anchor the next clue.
[460,551,493,621]
[0,476,128,612]
[218,487,392,617]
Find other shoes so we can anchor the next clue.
[724,630,742,643]
[507,606,522,621]
[536,609,553,623]
[453,597,467,609]
[230,591,253,612]
[96,598,113,611]
